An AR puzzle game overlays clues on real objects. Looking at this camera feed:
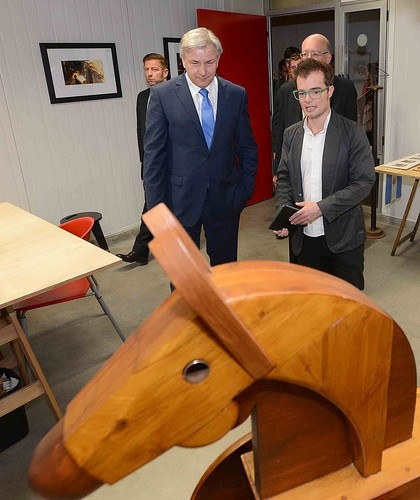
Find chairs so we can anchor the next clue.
[14,216,126,359]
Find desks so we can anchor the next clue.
[0,201,123,433]
[375,152,420,256]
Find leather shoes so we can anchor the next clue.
[117,252,149,263]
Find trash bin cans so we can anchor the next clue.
[0,367,29,453]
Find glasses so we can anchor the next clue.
[293,85,330,100]
[300,52,328,59]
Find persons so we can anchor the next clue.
[147,27,259,296]
[116,53,169,263]
[271,33,375,292]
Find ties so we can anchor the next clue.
[199,89,216,147]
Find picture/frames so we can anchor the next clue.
[38,37,123,106]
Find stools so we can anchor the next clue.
[60,208,112,249]
